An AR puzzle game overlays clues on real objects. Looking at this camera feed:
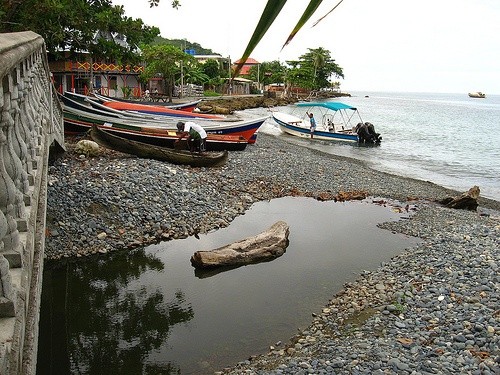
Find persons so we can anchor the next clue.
[145,88,150,97]
[153,88,158,96]
[174,122,207,156]
[306,112,317,138]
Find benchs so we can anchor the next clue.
[143,92,168,103]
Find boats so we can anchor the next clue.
[272,101,383,144]
[56,89,273,151]
[468,91,486,98]
[89,122,229,168]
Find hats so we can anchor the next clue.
[177,121,184,129]
